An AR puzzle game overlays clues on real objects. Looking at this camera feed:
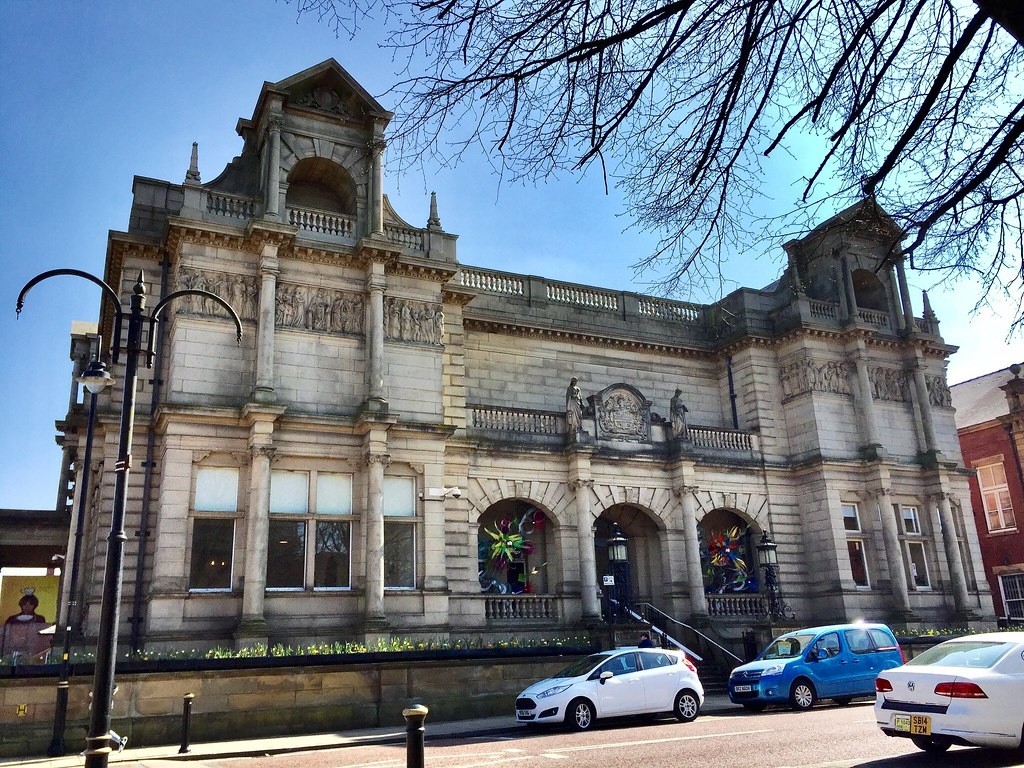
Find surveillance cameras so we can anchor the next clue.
[52,556,59,564]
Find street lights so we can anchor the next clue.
[45,333,117,758]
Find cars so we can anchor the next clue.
[872,630,1023,754]
[514,647,705,730]
[728,624,906,711]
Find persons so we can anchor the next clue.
[171,268,446,347]
[79,681,129,756]
[2,595,45,658]
[777,358,953,407]
[566,376,587,434]
[670,386,689,441]
[638,632,655,648]
